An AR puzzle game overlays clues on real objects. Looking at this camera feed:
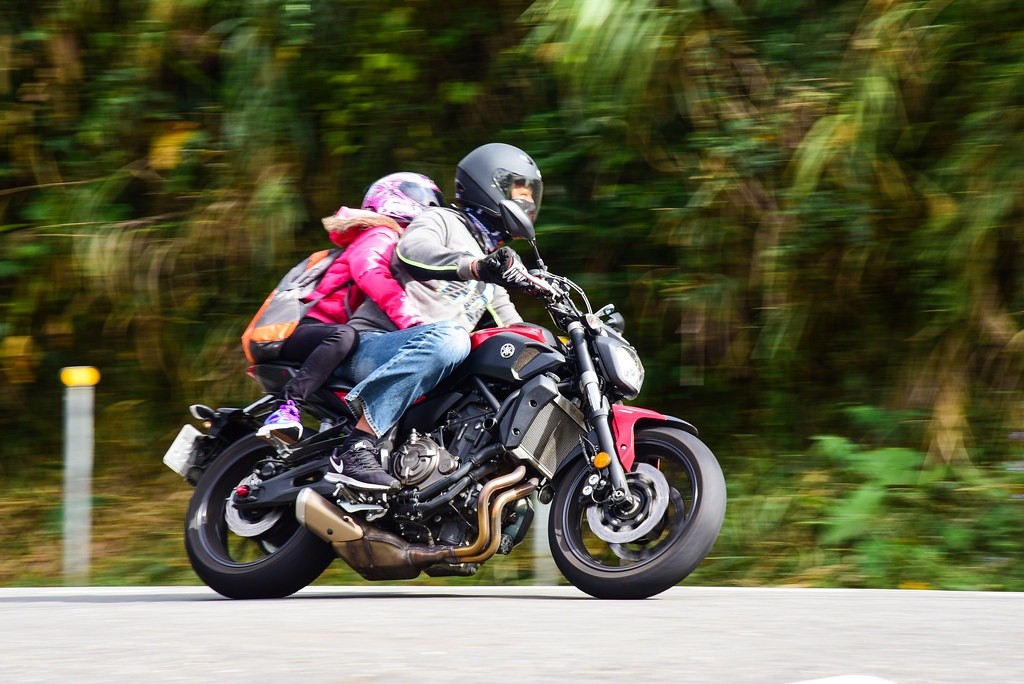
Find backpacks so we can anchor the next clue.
[239,243,346,366]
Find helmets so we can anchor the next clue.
[361,170,446,223]
[454,141,544,225]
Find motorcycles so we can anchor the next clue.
[161,257,726,601]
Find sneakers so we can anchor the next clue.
[323,439,402,494]
[257,399,304,446]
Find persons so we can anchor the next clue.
[249,142,544,491]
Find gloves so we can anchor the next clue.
[476,245,531,291]
